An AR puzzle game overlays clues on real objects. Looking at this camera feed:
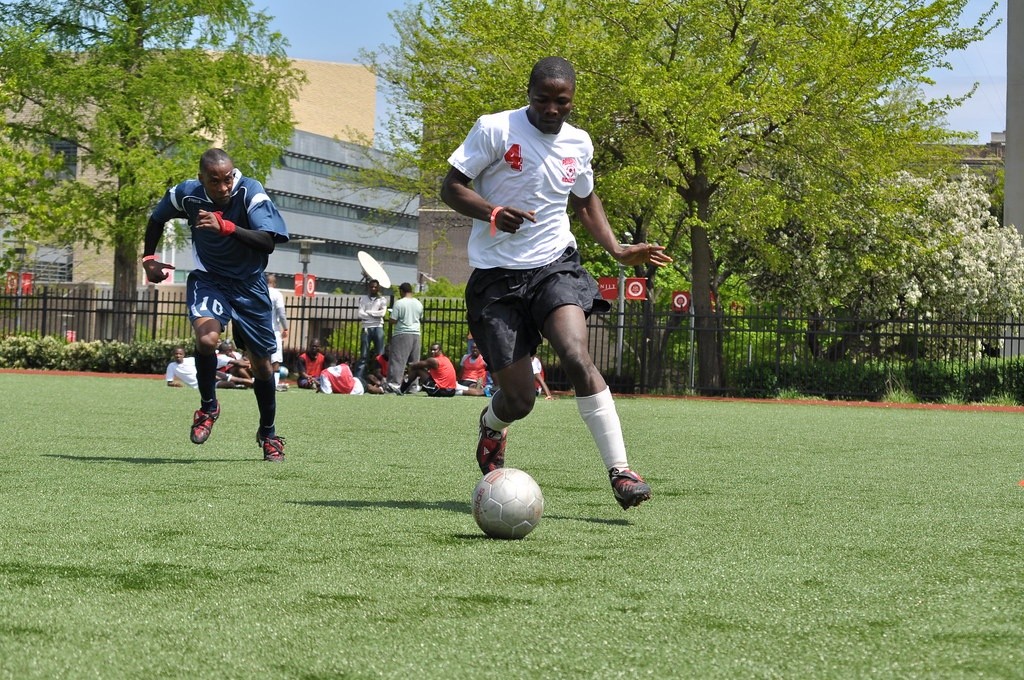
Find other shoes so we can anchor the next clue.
[455,390,462,395]
[388,383,403,396]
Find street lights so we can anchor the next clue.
[3,235,39,336]
[288,239,327,353]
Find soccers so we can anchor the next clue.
[471,467,545,539]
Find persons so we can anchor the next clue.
[296,338,555,399]
[441,57,672,510]
[385,282,424,396]
[143,148,289,462]
[357,280,387,378]
[266,275,288,392]
[166,338,289,392]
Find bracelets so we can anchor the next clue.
[142,255,160,262]
[490,206,504,237]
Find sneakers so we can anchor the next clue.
[476,407,508,476]
[609,469,651,510]
[256,430,286,462]
[190,401,221,444]
